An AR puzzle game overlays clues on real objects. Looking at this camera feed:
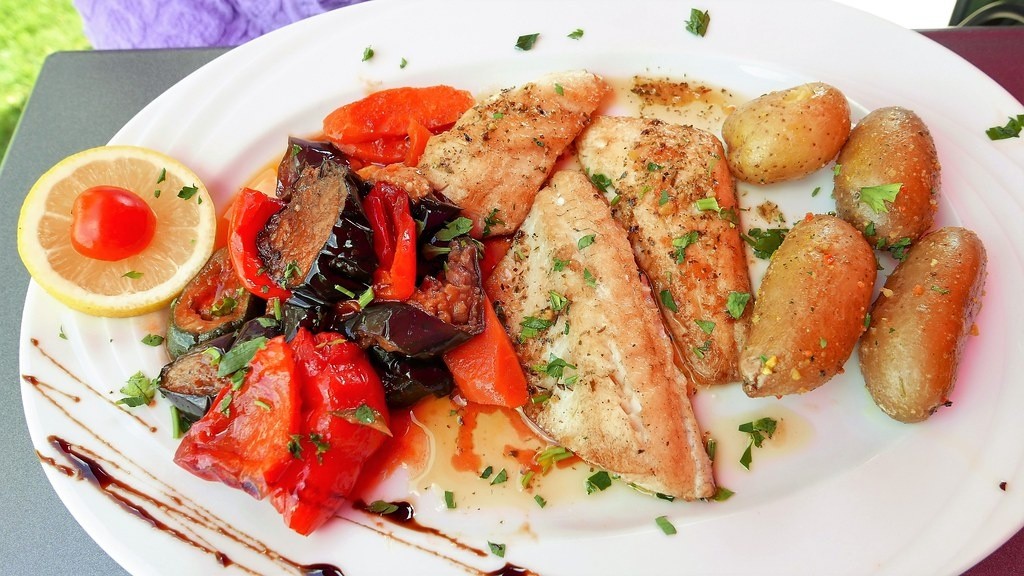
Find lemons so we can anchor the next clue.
[18,147,216,317]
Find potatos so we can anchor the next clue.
[739,213,876,397]
[834,106,941,249]
[720,81,851,184]
[859,226,988,423]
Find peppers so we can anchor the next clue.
[175,325,390,536]
[228,188,291,302]
[366,180,416,301]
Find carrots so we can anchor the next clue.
[442,288,527,407]
[324,85,473,143]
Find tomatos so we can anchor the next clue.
[71,186,157,261]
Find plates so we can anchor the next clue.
[20,0,1024,576]
